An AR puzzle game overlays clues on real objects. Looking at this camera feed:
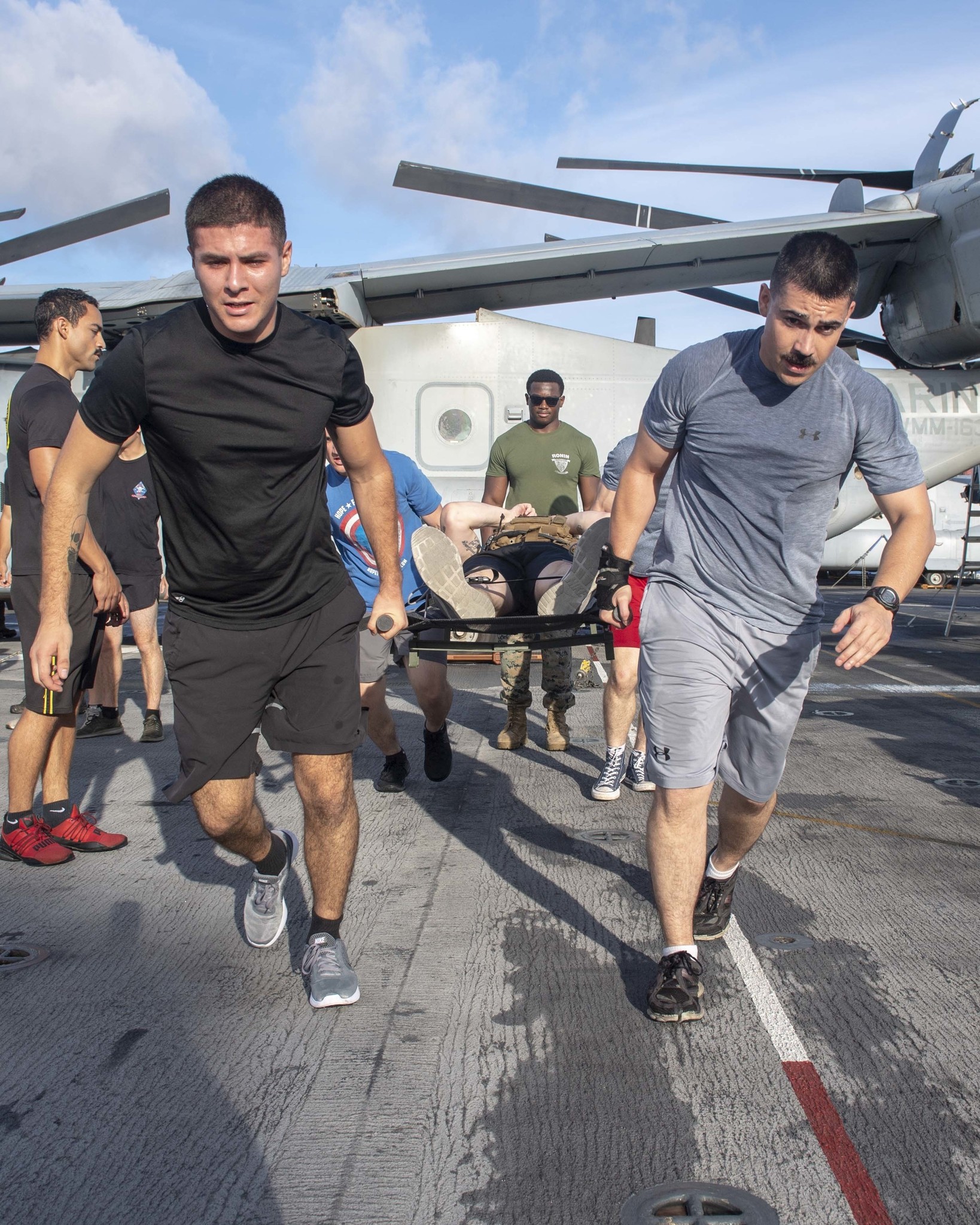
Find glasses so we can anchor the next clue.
[525,392,562,407]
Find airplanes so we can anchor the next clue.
[0,95,979,589]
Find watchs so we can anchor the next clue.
[864,586,898,620]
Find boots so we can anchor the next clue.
[496,709,528,749]
[544,709,571,751]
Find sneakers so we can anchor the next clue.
[75,712,124,738]
[693,841,738,941]
[423,718,453,783]
[625,749,657,792]
[5,719,20,730]
[647,949,707,1022]
[81,706,102,730]
[375,749,411,794]
[139,715,165,742]
[50,803,128,853]
[10,695,27,714]
[75,700,87,717]
[0,811,75,867]
[301,931,360,1008]
[243,829,300,948]
[591,747,626,800]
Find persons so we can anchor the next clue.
[596,233,931,1024]
[0,429,168,742]
[587,431,679,799]
[317,425,454,792]
[0,290,126,868]
[30,173,408,1008]
[410,501,611,633]
[481,370,599,751]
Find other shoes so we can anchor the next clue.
[535,515,610,626]
[411,526,497,631]
